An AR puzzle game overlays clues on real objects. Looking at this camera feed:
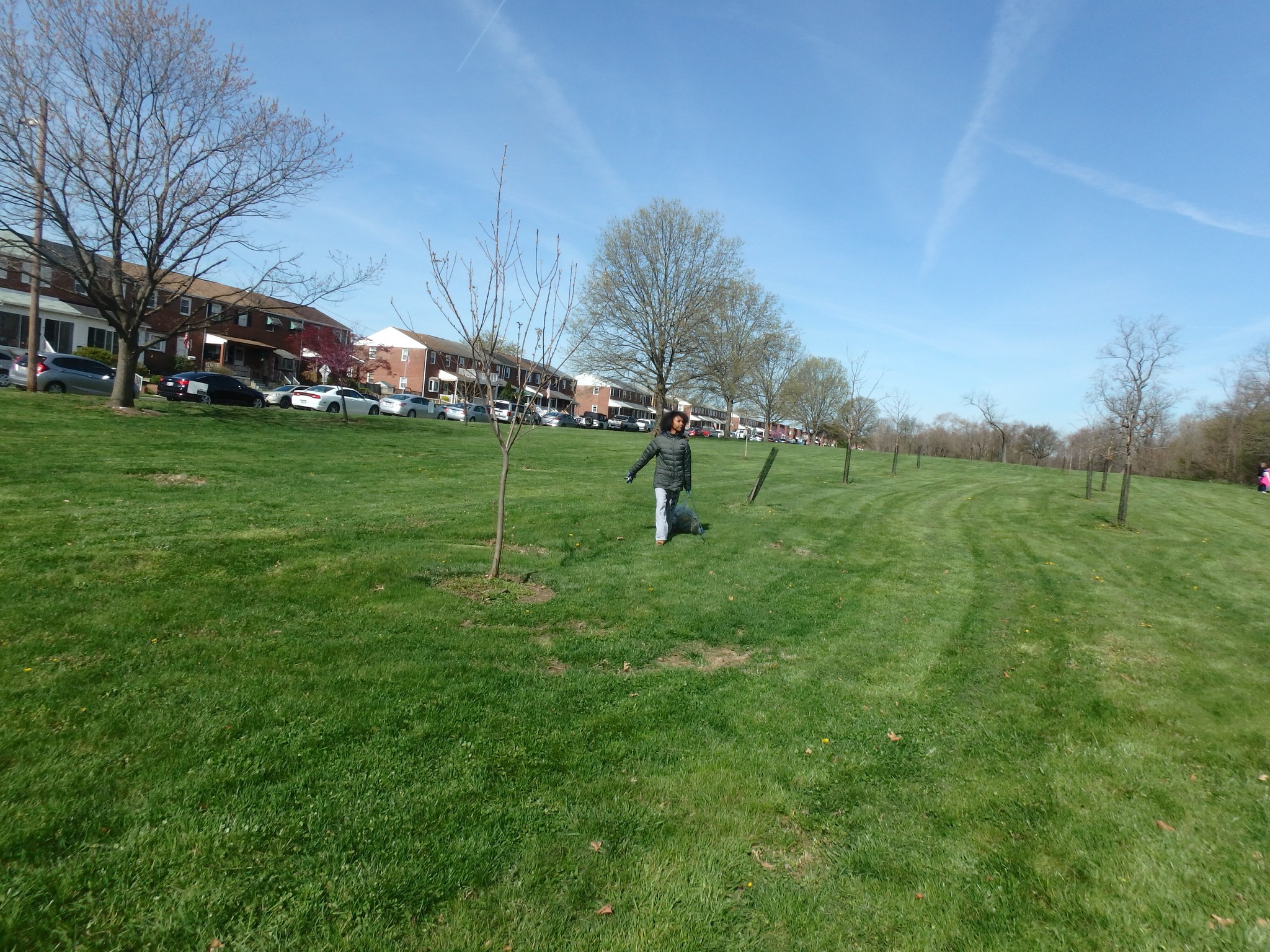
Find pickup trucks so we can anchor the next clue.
[610,414,640,432]
[635,419,655,433]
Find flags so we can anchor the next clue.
[183,327,190,349]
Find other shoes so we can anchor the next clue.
[655,540,665,546]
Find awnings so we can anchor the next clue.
[289,321,305,331]
[266,316,283,326]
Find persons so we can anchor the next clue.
[803,433,808,445]
[623,412,691,545]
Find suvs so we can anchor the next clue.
[578,411,609,430]
[489,399,539,425]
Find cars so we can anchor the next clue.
[262,385,325,409]
[700,427,718,437]
[540,412,580,429]
[440,404,465,409]
[730,430,763,441]
[768,436,803,444]
[575,418,581,426]
[156,372,266,408]
[715,430,725,438]
[0,349,21,387]
[683,428,690,436]
[687,428,705,437]
[380,394,448,420]
[291,385,381,415]
[8,352,140,399]
[445,403,493,422]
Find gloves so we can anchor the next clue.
[624,471,637,484]
